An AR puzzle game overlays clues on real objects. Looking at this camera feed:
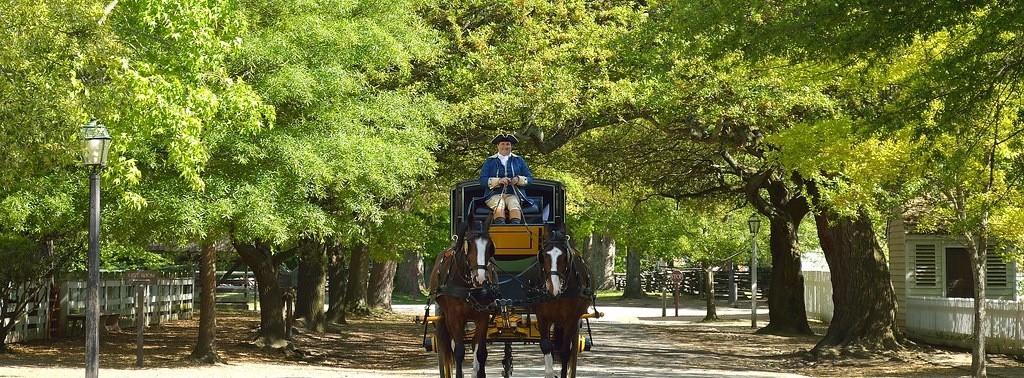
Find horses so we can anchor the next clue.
[523,222,596,378]
[429,213,500,378]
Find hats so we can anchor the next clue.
[491,133,518,144]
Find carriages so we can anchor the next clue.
[410,176,605,378]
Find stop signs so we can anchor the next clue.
[671,270,683,283]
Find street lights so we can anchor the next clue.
[746,212,762,329]
[78,117,113,378]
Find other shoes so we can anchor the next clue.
[494,218,505,224]
[510,219,520,225]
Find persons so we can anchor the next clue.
[478,134,534,224]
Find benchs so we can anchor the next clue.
[471,195,544,224]
[68,312,122,339]
